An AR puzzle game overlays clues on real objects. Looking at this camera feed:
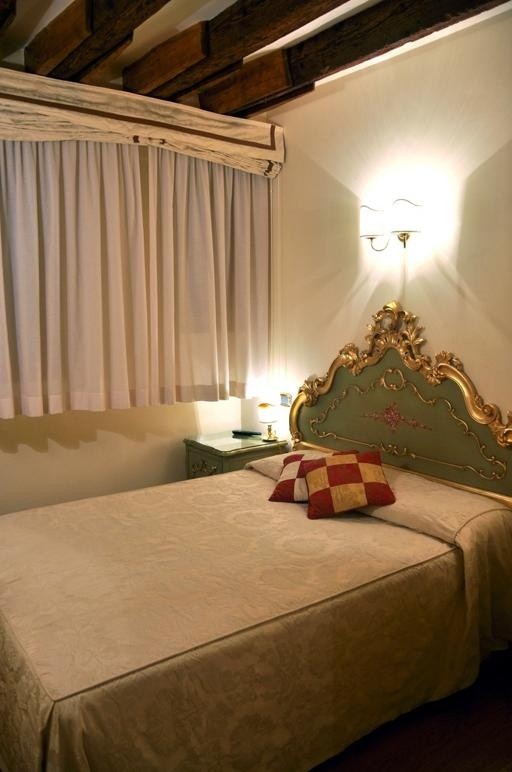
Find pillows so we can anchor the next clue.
[301,451,395,519]
[268,449,359,503]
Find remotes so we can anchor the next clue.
[232,429,262,437]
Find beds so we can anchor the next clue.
[1,300,512,772]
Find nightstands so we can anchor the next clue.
[183,429,288,479]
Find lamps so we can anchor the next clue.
[257,402,279,441]
[358,198,421,251]
